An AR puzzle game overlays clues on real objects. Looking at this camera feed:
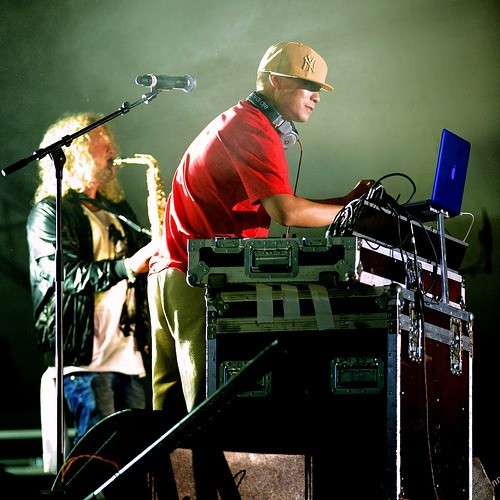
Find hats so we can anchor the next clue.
[258,42,334,92]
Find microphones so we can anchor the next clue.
[135,74,196,94]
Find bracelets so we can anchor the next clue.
[124,258,136,283]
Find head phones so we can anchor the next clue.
[246,90,299,150]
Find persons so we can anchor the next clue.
[147,41,377,413]
[26,112,160,445]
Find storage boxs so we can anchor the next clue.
[188,238,466,309]
[205,283,473,500]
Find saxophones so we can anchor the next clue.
[108,154,166,239]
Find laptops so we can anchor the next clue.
[399,128,471,223]
